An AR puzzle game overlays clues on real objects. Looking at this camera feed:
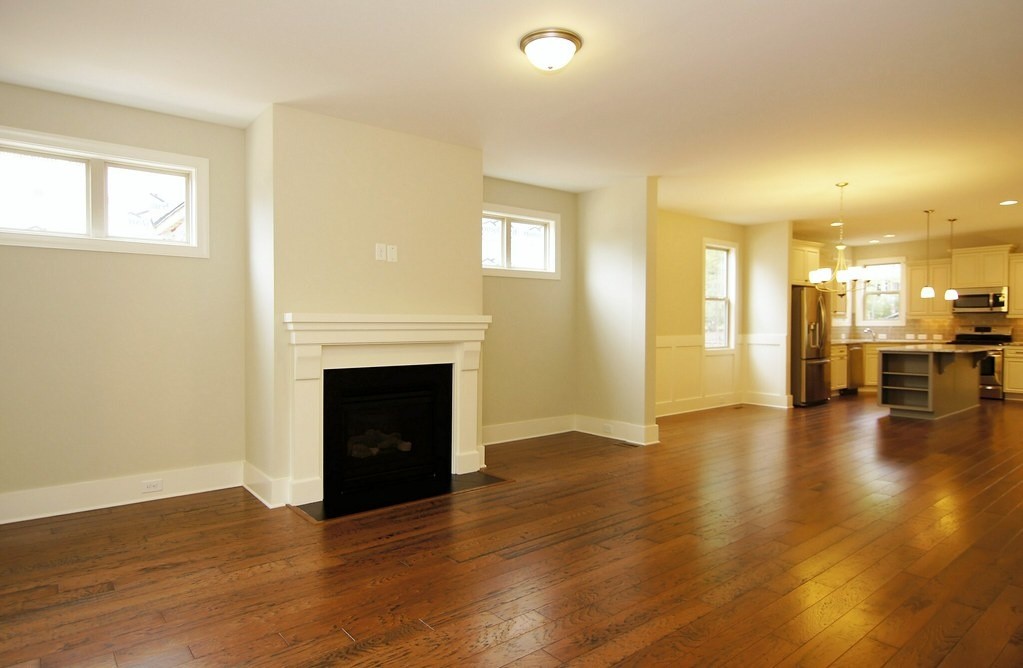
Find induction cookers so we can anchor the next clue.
[946,325,1013,345]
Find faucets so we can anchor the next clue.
[863,327,876,341]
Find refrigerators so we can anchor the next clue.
[791,283,832,406]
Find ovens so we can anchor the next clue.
[979,351,1003,398]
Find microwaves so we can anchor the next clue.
[952,286,1009,313]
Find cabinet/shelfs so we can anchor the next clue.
[829,344,1023,420]
[907,246,1023,318]
[792,238,823,286]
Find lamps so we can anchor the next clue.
[519,26,582,71]
[807,181,871,297]
[921,210,935,297]
[944,218,960,300]
